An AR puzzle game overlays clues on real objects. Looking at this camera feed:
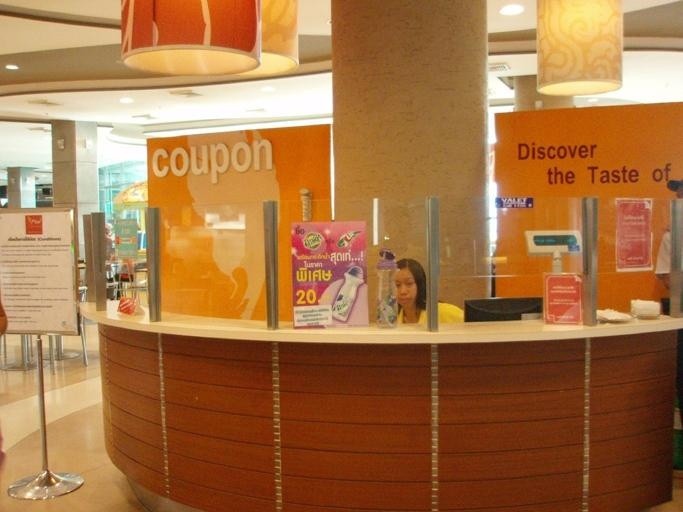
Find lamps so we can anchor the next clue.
[121,1,301,80]
[535,0,622,98]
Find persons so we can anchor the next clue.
[389,258,465,323]
[652,179,682,301]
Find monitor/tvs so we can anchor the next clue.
[464,296,543,322]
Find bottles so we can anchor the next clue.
[374,250,398,328]
[332,267,365,322]
